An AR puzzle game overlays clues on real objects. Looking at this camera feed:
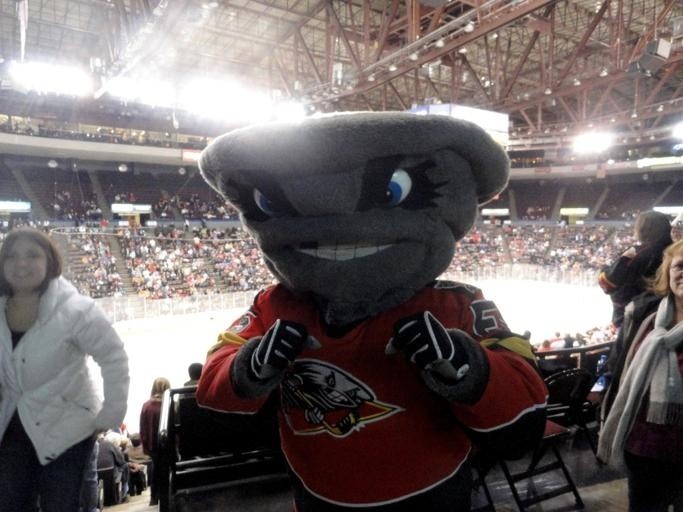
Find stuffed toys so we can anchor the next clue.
[194,112,548,511]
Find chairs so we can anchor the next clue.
[472,368,596,511]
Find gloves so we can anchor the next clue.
[386,308,455,372]
[249,318,323,379]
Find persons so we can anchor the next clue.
[0,111,281,299]
[87,362,217,512]
[1,231,130,512]
[445,122,683,511]
